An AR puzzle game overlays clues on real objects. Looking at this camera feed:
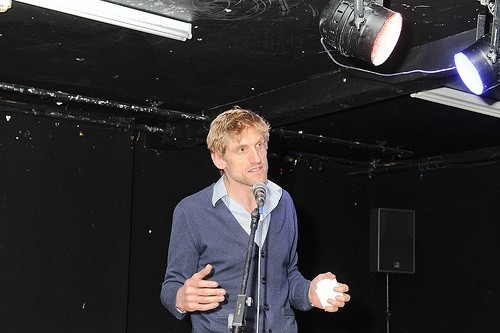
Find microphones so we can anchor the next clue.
[252,182,268,221]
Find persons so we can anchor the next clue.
[159,106,351,333]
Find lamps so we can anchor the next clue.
[320,0,403,66]
[8,0,192,42]
[454,0,500,96]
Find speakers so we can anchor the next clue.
[370,209,416,273]
